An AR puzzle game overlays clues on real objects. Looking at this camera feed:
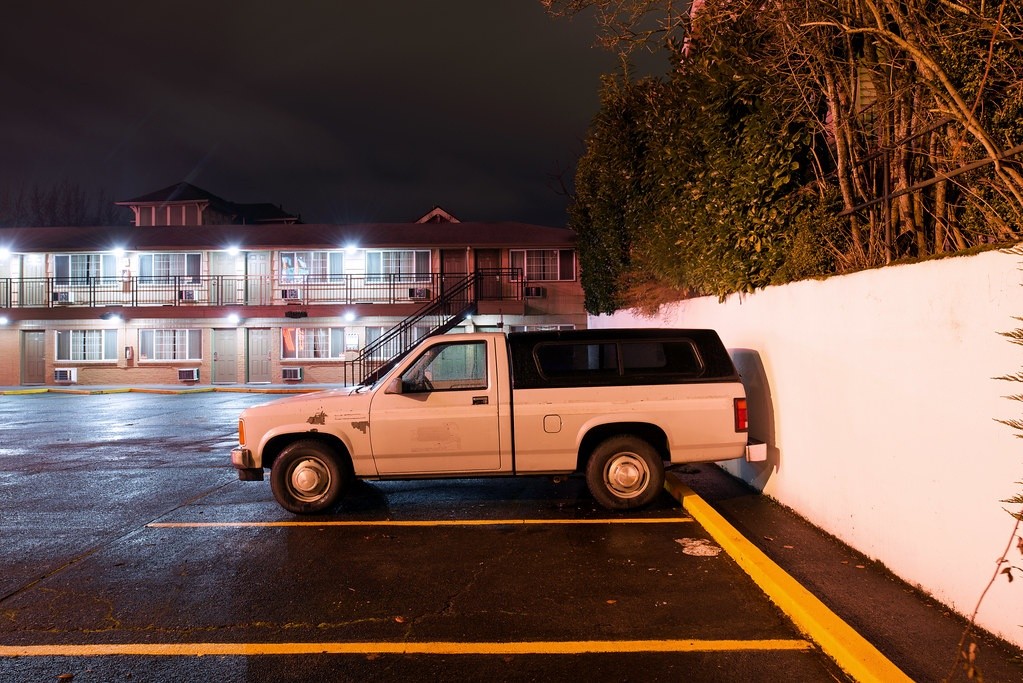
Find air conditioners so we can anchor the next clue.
[177,368,200,381]
[281,288,301,300]
[53,292,74,303]
[178,289,197,303]
[54,368,77,383]
[282,368,302,380]
[524,286,544,298]
[409,287,427,298]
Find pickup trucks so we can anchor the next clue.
[228,327,766,517]
